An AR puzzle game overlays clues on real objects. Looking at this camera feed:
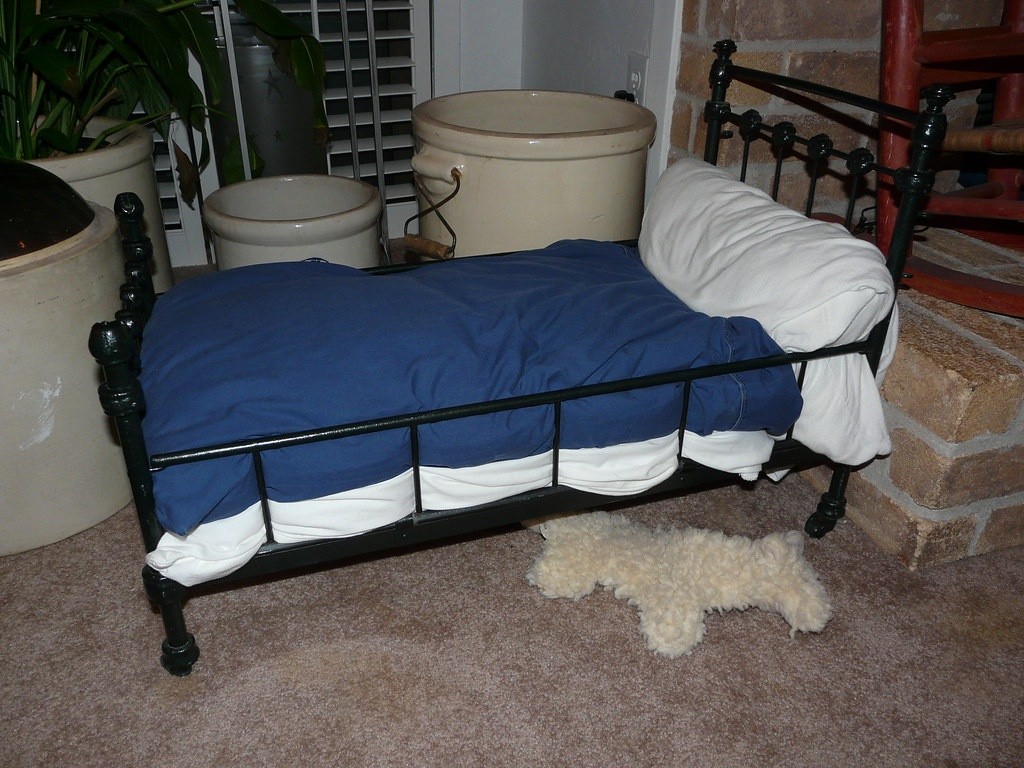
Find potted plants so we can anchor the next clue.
[0,0,329,298]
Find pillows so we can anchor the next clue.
[642,159,899,468]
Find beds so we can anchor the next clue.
[85,34,958,678]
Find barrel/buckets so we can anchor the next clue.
[402,89,658,264]
[203,175,390,270]
